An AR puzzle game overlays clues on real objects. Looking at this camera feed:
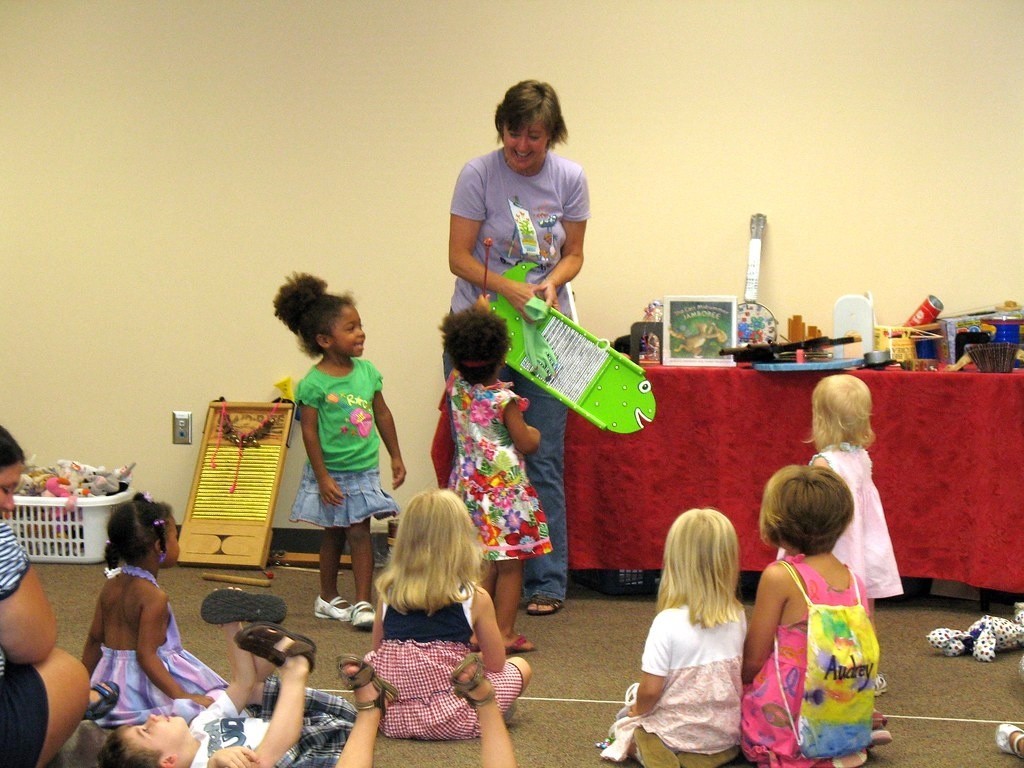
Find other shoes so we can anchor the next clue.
[314,592,356,622]
[352,602,375,627]
[634,726,680,768]
[995,723,1024,757]
[679,743,740,768]
[201,589,287,623]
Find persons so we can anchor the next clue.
[97,586,357,768]
[363,490,532,740]
[273,274,406,627]
[0,425,120,768]
[334,654,517,768]
[441,308,554,654]
[739,465,894,768]
[802,374,904,695]
[81,491,230,729]
[234,622,399,768]
[442,80,593,615]
[995,723,1024,759]
[594,508,747,768]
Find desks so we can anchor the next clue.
[429,365,1024,613]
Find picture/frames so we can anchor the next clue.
[663,294,737,367]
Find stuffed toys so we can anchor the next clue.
[13,460,136,554]
[925,602,1024,663]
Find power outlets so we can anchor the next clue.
[171,410,192,445]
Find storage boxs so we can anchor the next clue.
[933,307,1024,365]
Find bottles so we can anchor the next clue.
[637,300,664,362]
[905,296,943,326]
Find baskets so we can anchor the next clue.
[2,488,137,564]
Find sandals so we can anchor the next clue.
[527,594,562,614]
[505,636,531,657]
[830,744,868,768]
[867,711,892,746]
[468,641,481,650]
[81,680,119,719]
[234,622,316,673]
[450,656,497,708]
[334,654,398,720]
